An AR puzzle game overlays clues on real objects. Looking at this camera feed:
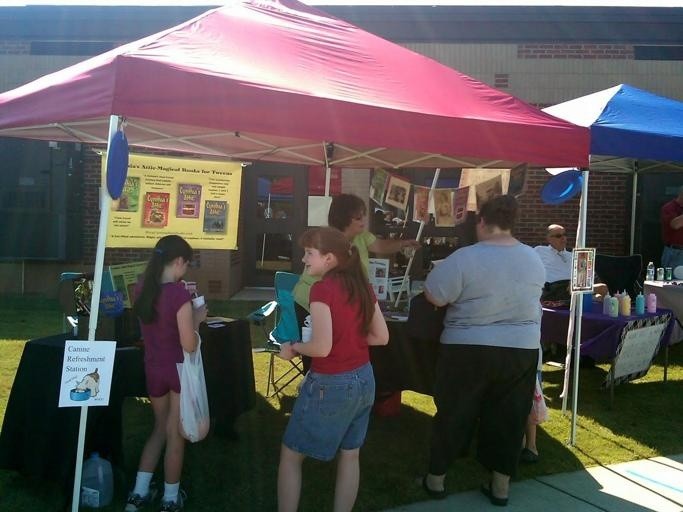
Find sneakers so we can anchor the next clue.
[125,482,160,512]
[161,488,187,510]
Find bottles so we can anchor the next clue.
[646,262,655,281]
[603,289,656,318]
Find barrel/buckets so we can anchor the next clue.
[79,452,115,509]
[373,392,405,418]
[115,307,141,342]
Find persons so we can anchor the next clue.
[660,185,683,268]
[417,196,546,506]
[520,346,548,462]
[533,224,608,368]
[293,194,422,374]
[125,235,209,512]
[277,227,389,512]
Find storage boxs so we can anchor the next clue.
[174,203,247,302]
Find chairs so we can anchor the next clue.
[246,249,451,443]
[540,249,682,410]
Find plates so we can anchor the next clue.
[674,265,683,280]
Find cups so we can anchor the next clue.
[192,294,206,307]
[302,327,312,343]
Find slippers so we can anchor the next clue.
[416,473,447,498]
[519,448,538,463]
[481,480,508,504]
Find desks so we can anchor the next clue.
[0,310,258,496]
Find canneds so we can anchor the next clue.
[665,267,673,281]
